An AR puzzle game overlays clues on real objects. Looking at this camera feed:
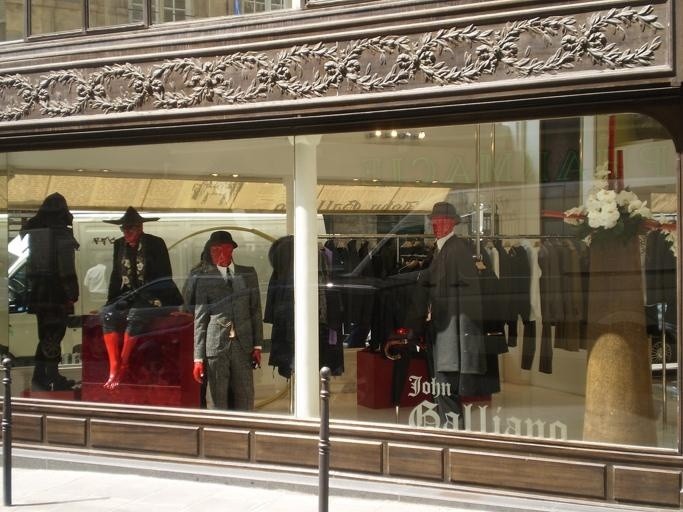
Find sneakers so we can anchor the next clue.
[32,377,77,393]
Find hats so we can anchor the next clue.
[426,202,461,226]
[205,232,237,250]
[103,205,160,225]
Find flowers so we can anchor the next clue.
[573,189,656,250]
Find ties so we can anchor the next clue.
[226,267,234,287]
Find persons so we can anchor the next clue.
[102,206,184,390]
[422,201,488,430]
[193,231,264,414]
[22,191,77,390]
